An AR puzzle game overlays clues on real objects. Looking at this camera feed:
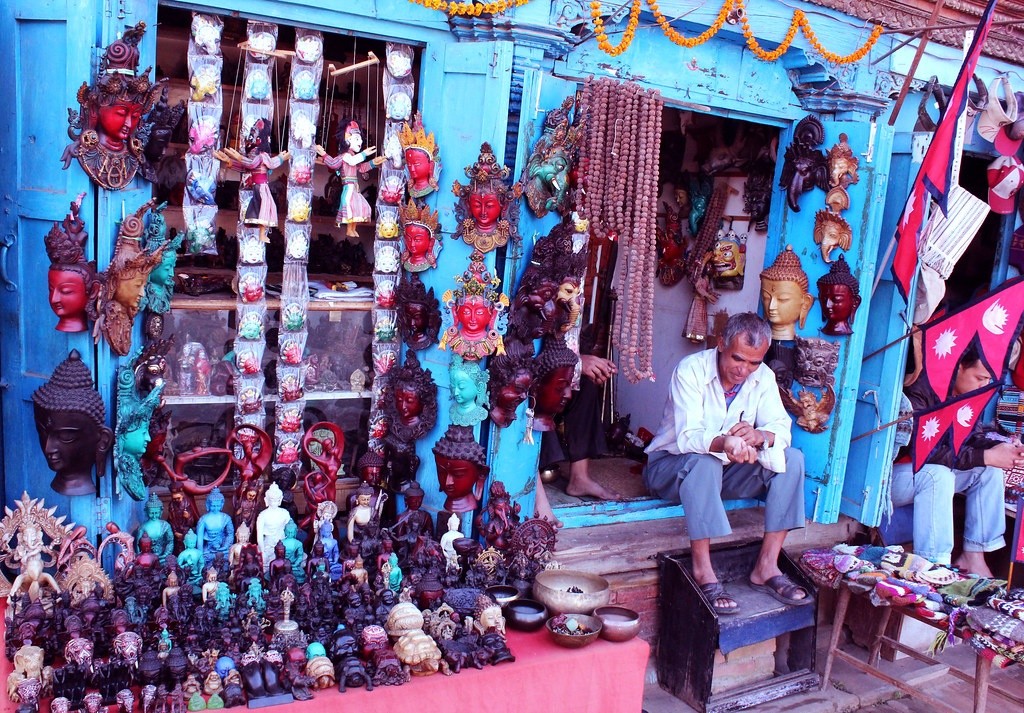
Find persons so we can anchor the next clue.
[445,106,588,428]
[0,422,552,713]
[760,117,862,432]
[644,313,816,613]
[892,350,1024,579]
[535,354,626,531]
[33,22,177,500]
[655,112,780,343]
[181,18,485,513]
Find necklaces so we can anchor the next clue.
[576,75,663,383]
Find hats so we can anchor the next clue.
[913,262,946,325]
[964,72,989,133]
[994,91,1024,156]
[913,74,947,131]
[803,538,1024,669]
[987,154,1024,214]
[977,76,1018,143]
[883,392,914,525]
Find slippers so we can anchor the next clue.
[748,572,815,606]
[699,582,740,614]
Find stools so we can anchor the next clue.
[821,578,1024,713]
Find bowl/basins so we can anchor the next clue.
[484,585,524,610]
[503,599,548,631]
[533,569,610,616]
[546,614,602,648]
[592,606,641,642]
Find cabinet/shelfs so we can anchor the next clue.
[153,77,382,524]
